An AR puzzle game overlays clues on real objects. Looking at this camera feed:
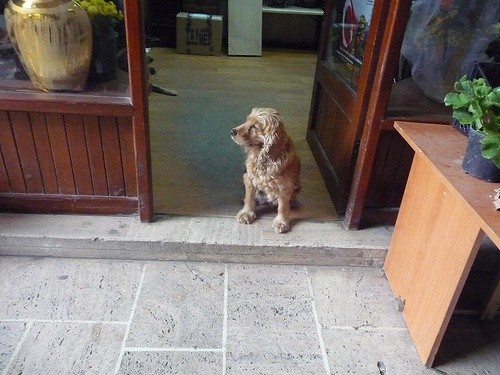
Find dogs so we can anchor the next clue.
[230,107,302,233]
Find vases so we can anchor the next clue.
[89,32,120,82]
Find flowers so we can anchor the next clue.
[75,0,124,38]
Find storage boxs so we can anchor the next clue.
[175,10,223,56]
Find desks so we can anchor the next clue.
[380,118,499,368]
[262,2,323,49]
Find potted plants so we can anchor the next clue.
[461,86,500,182]
[442,72,492,137]
